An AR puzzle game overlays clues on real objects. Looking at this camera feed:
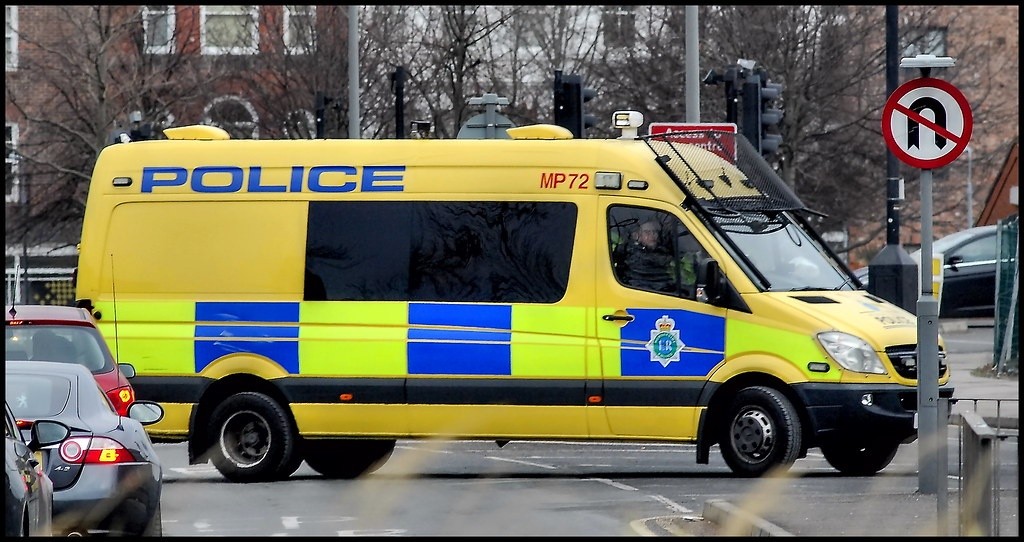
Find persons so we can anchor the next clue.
[330,231,408,300]
[619,216,696,297]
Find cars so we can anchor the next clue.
[6,304,137,419]
[5,401,72,537]
[6,361,163,537]
[848,222,1020,317]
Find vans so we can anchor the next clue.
[71,125,955,482]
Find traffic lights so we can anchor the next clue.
[744,71,784,160]
